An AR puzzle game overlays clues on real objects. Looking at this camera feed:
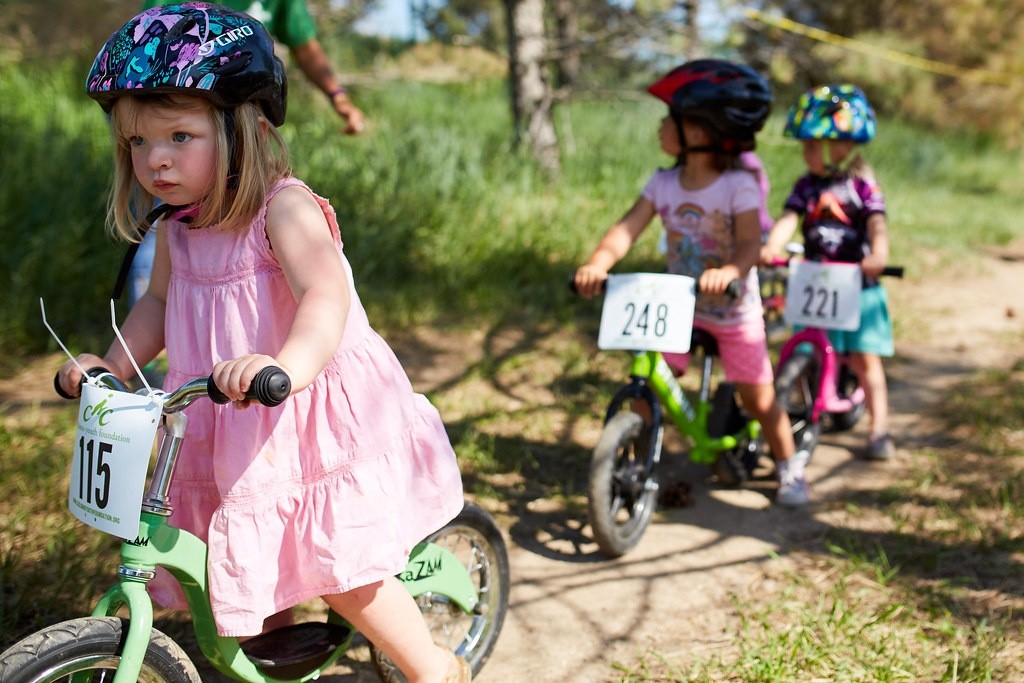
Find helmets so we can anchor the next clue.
[780,84,876,142]
[644,59,771,140]
[85,2,286,127]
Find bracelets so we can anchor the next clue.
[329,89,347,98]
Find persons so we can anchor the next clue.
[140,0,368,136]
[760,83,897,462]
[574,60,815,505]
[58,2,472,683]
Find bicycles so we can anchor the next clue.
[566,242,905,557]
[0,364,512,683]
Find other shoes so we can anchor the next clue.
[775,460,810,505]
[865,432,890,459]
